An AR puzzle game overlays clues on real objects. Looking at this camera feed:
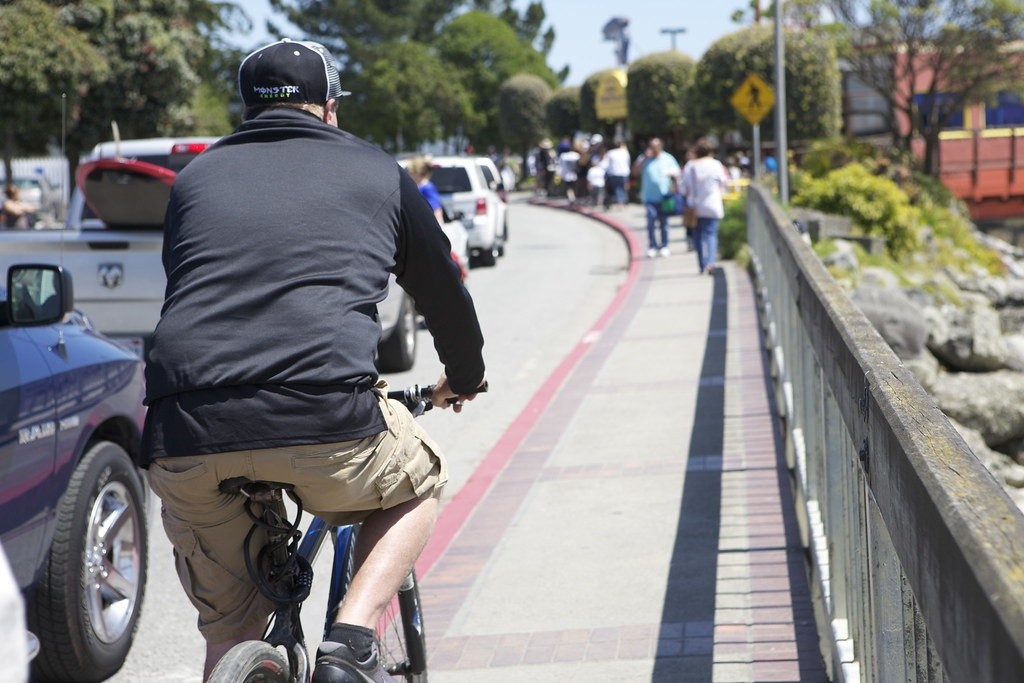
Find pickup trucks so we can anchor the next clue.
[0,137,416,373]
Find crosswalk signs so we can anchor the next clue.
[729,73,774,125]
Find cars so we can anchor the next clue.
[0,262,149,683]
[396,158,507,277]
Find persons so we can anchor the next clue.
[636,136,681,257]
[721,145,802,182]
[3,184,44,231]
[682,141,729,278]
[531,129,633,211]
[137,35,493,675]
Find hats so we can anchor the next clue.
[238,38,353,107]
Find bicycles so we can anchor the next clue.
[206,381,488,683]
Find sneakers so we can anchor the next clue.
[311,641,393,683]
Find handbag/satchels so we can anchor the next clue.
[683,207,698,228]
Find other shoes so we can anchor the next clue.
[648,246,657,258]
[660,246,668,257]
